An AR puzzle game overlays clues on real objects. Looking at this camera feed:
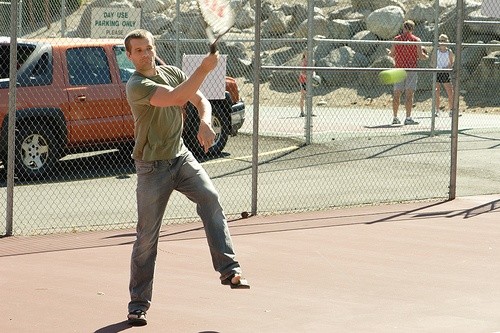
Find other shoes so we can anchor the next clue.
[300,112,307,117]
[434,111,440,117]
[404,118,419,125]
[392,118,401,124]
[449,111,462,117]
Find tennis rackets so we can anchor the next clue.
[196,0,237,54]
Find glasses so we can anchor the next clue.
[438,40,444,42]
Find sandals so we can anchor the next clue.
[128,308,148,325]
[221,272,250,289]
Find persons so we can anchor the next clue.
[299,48,318,117]
[125,29,250,324]
[390,19,429,124]
[431,34,454,118]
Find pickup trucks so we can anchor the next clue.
[0,36,247,181]
